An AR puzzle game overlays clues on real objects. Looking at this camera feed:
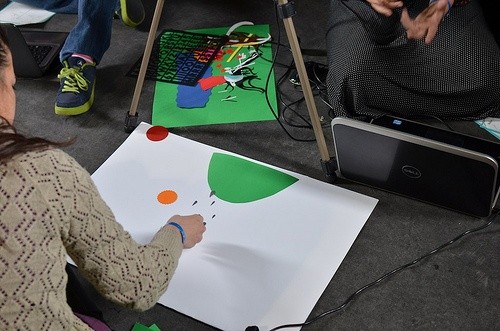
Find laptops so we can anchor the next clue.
[331,112,500,219]
[0,22,69,79]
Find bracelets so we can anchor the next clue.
[430,0,451,10]
[167,221,186,243]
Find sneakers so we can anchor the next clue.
[55,55,97,115]
[114,0,145,25]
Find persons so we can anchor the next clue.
[9,0,147,116]
[0,38,206,331]
[324,0,500,121]
